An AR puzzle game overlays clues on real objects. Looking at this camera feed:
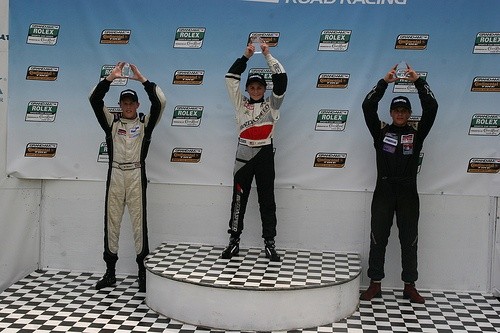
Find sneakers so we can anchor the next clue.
[222,243,240,258]
[403,283,424,303]
[359,283,381,300]
[96,273,116,288]
[265,246,279,262]
[137,272,145,290]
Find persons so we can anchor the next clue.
[87,62,167,292]
[360,61,439,304]
[222,43,289,261]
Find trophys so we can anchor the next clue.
[120,63,134,78]
[392,59,411,78]
[250,35,264,53]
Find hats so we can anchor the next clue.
[245,73,267,88]
[390,96,411,110]
[120,89,138,101]
